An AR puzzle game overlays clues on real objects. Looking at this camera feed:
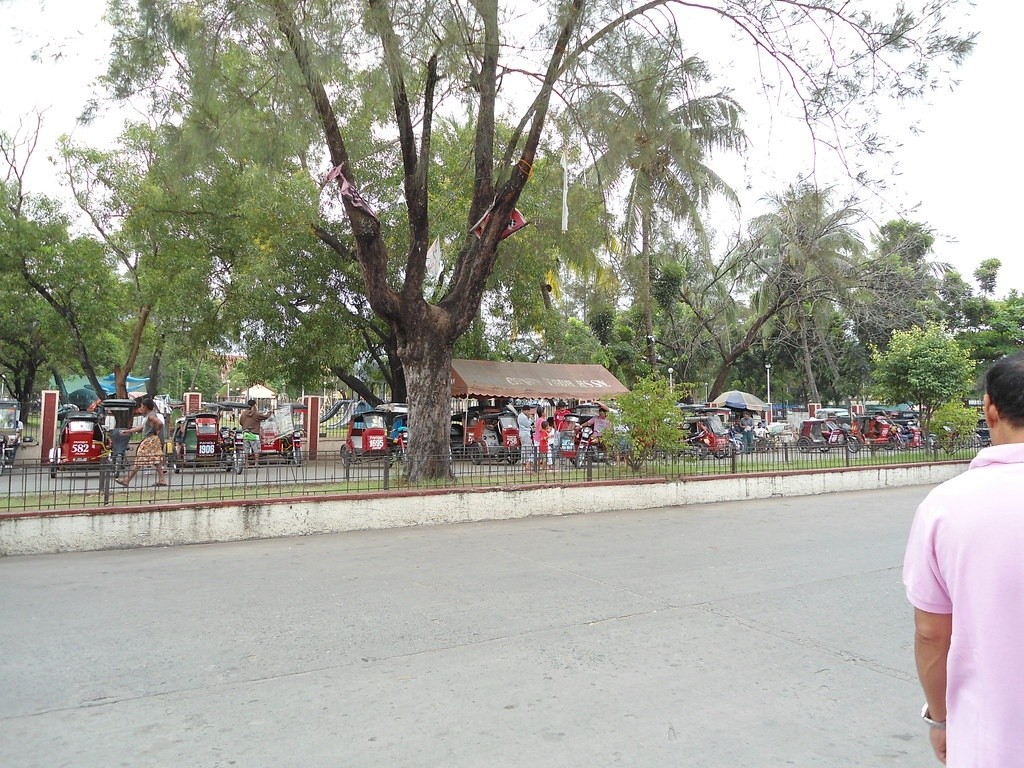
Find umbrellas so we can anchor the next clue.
[710,390,770,412]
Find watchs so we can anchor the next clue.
[921,701,946,729]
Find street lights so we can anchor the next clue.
[668,367,674,393]
[705,382,708,402]
[1,374,6,395]
[765,363,772,403]
[189,380,328,413]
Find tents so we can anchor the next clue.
[445,358,630,456]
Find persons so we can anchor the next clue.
[553,401,575,431]
[538,420,558,470]
[3,412,24,468]
[575,408,612,465]
[115,398,167,487]
[517,405,536,470]
[525,407,548,470]
[614,435,632,467]
[831,416,921,448]
[546,422,556,466]
[901,351,1024,767]
[738,412,756,454]
[240,400,275,469]
[755,422,769,443]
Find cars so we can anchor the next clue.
[169,398,215,409]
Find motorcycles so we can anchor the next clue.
[340,410,408,465]
[449,398,992,469]
[48,412,122,478]
[261,404,306,466]
[0,398,23,478]
[173,411,243,475]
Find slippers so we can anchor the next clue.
[152,482,167,487]
[115,478,129,487]
